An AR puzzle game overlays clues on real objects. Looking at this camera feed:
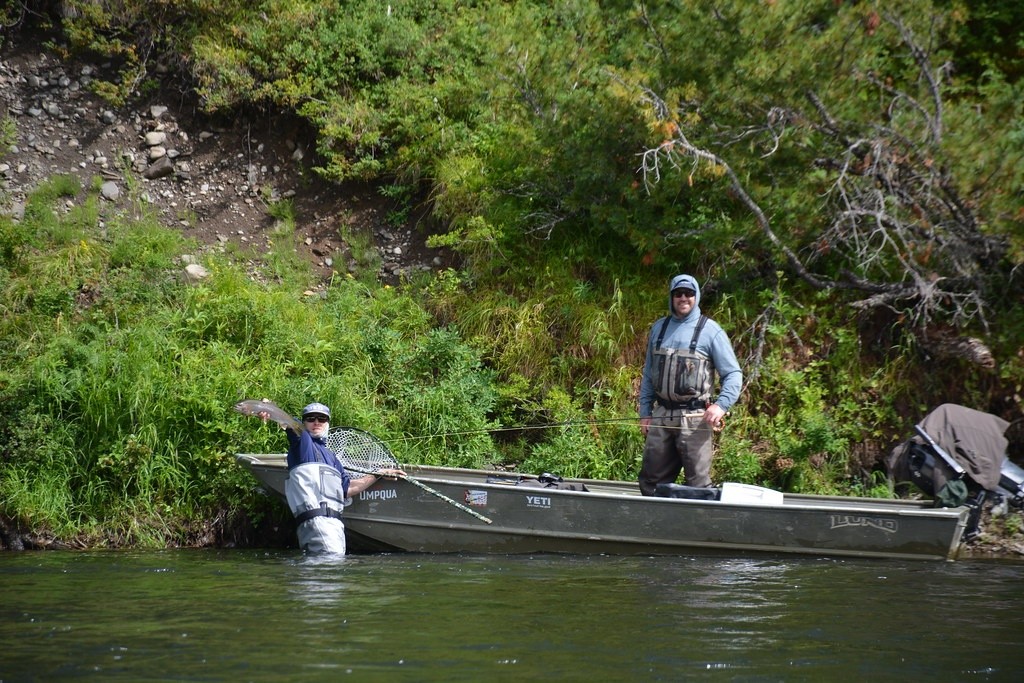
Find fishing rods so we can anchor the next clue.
[385,411,731,443]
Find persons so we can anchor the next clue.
[257,397,409,556]
[637,273,744,496]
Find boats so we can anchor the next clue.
[234,453,973,566]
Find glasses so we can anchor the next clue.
[671,287,696,297]
[303,415,329,423]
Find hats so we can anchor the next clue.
[673,280,696,291]
[302,403,330,418]
